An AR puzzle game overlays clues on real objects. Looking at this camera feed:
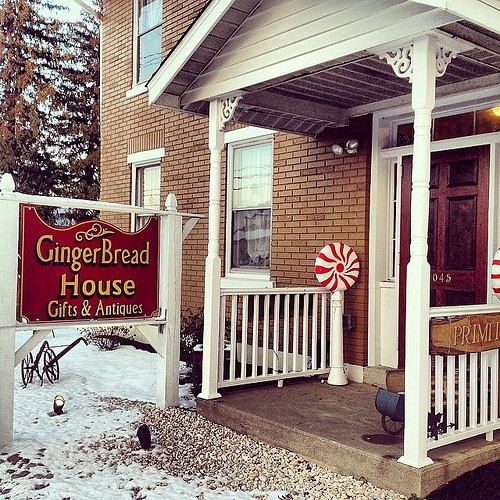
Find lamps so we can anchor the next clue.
[54,394,66,416]
[135,424,152,451]
[330,138,360,157]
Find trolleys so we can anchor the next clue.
[21,337,89,388]
